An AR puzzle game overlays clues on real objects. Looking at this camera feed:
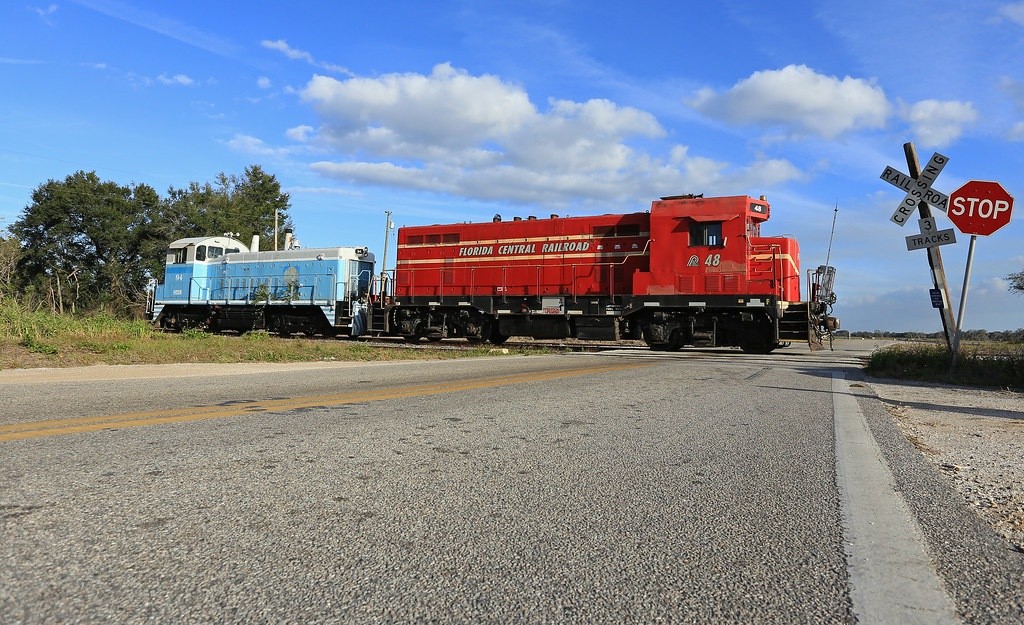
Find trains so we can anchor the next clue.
[149,192,846,354]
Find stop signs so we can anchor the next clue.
[945,179,1016,237]
